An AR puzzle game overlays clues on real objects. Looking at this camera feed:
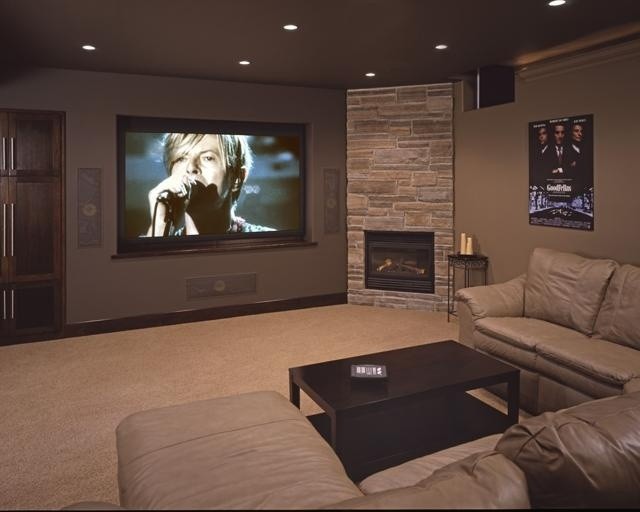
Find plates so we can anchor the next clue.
[456,252,476,257]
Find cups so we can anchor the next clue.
[460,232,473,255]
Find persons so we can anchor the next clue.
[138,132,278,238]
[538,123,584,182]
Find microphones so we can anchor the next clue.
[158,183,194,202]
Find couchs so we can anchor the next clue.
[453,246,640,418]
[116,390,640,512]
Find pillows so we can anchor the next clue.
[592,263,640,351]
[524,247,620,338]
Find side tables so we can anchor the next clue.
[447,252,490,322]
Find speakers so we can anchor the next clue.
[462,65,514,113]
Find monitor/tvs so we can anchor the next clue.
[118,117,305,246]
